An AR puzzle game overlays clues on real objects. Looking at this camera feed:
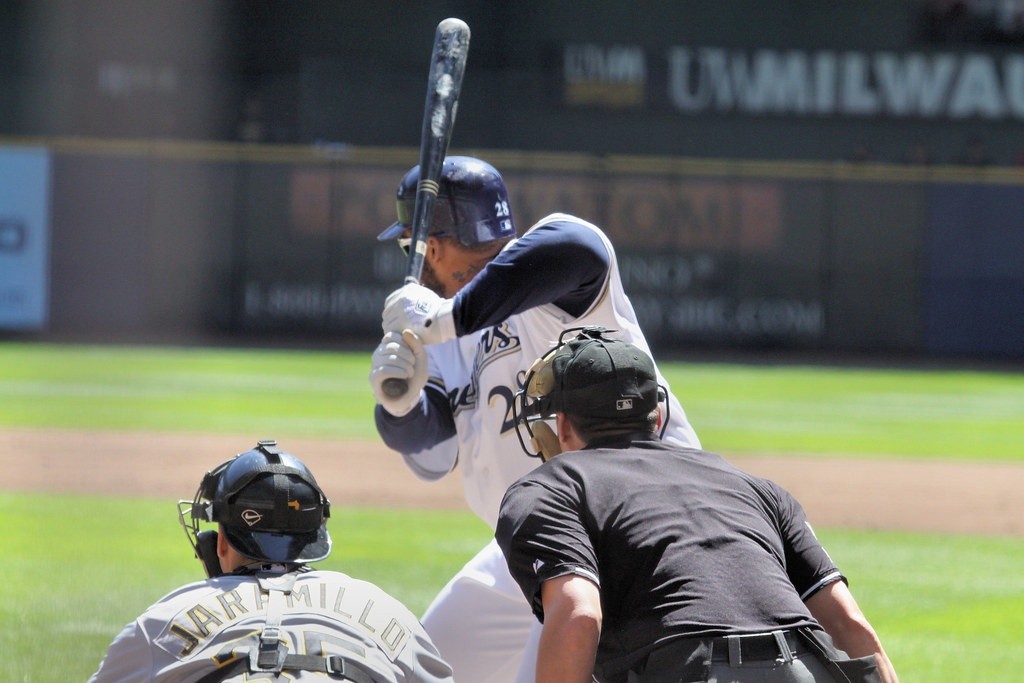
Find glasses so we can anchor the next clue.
[396,232,451,257]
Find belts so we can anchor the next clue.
[629,630,810,676]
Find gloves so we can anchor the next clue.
[381,283,457,345]
[369,329,427,417]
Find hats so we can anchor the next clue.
[540,333,658,419]
[215,449,332,563]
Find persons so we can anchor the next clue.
[368,155,701,683]
[89,440,455,683]
[495,326,899,683]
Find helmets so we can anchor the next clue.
[377,156,517,246]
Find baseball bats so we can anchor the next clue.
[378,16,474,402]
